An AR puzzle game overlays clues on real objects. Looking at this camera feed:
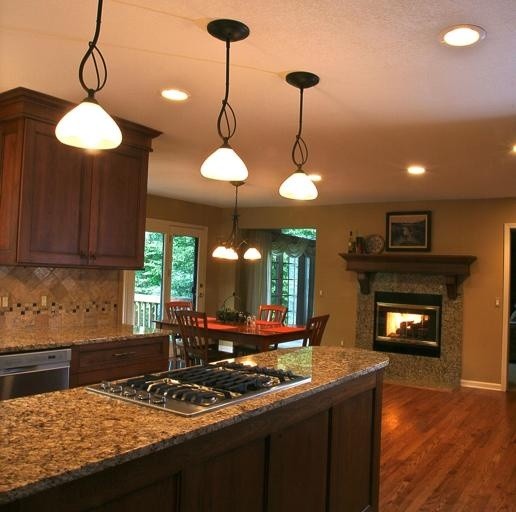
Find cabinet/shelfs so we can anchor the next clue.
[67,333,170,389]
[0,85,165,270]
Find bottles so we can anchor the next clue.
[247,315,256,327]
[346,230,365,253]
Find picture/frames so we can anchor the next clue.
[384,210,433,252]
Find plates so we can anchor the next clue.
[365,234,384,254]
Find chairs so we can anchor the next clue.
[149,297,332,369]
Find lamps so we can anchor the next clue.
[277,70,322,201]
[212,180,262,261]
[197,20,250,183]
[53,1,124,151]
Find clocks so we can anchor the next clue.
[366,234,386,255]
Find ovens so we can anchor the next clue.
[0,362,69,400]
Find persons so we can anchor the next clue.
[400,225,419,245]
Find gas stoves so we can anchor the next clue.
[85,361,311,416]
